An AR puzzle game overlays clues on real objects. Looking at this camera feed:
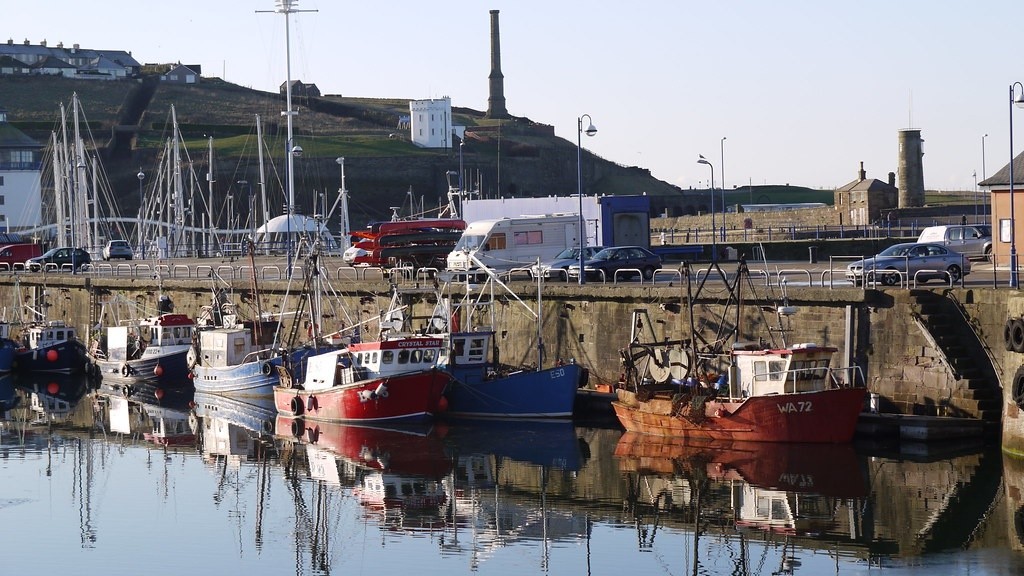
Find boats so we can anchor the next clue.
[272,283,456,425]
[0,320,15,376]
[186,236,340,394]
[445,418,586,531]
[611,255,868,442]
[274,414,452,533]
[17,320,87,375]
[86,383,198,447]
[14,375,90,428]
[85,277,195,386]
[313,250,582,424]
[611,430,870,576]
[192,389,305,549]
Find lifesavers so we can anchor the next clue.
[121,364,130,377]
[1003,319,1024,353]
[122,384,131,397]
[262,419,273,434]
[290,397,304,416]
[291,418,304,437]
[262,361,273,377]
[1011,364,1024,411]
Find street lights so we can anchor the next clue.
[286,138,466,278]
[578,114,727,284]
[972,81,1024,288]
[71,154,145,274]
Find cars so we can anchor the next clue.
[844,225,992,285]
[343,210,662,283]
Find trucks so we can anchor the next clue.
[0,240,132,272]
